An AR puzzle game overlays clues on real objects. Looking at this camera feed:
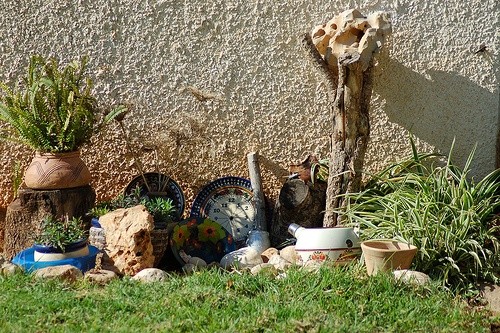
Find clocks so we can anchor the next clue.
[204,192,257,241]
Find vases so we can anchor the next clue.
[360,239,418,278]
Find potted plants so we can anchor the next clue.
[85,185,180,267]
[0,51,130,191]
[26,211,91,262]
